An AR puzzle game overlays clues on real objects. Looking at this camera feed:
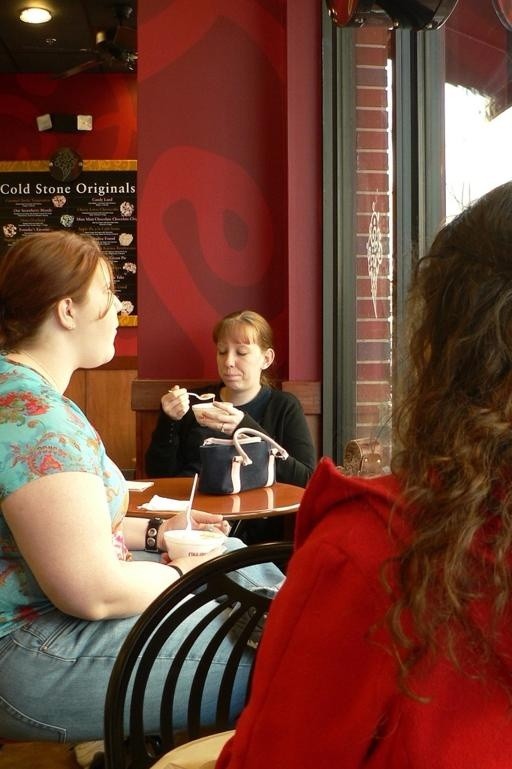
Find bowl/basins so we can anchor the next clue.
[162,530,225,561]
[191,402,233,426]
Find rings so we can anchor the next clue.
[220,422,224,433]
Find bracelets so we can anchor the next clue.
[142,517,163,554]
[168,562,183,577]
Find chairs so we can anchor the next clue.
[104,539,295,768]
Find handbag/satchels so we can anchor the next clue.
[199,427,288,494]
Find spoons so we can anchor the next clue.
[168,390,215,401]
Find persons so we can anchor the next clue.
[0,231,255,743]
[144,310,314,546]
[217,178,512,769]
